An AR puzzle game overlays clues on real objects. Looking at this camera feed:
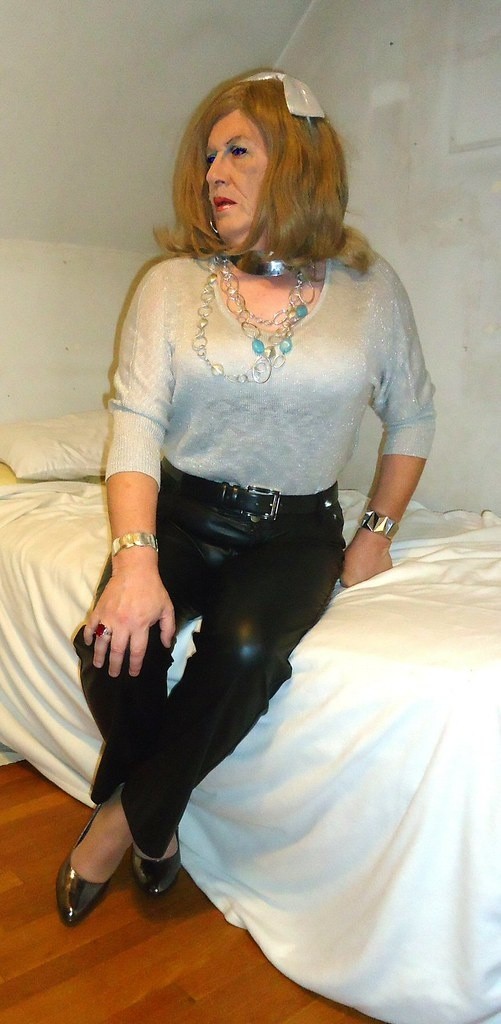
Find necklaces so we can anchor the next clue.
[231,250,288,277]
[193,256,315,384]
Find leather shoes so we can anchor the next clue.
[131,825,180,895]
[56,803,114,927]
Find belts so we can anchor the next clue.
[160,457,339,520]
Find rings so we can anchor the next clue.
[96,624,112,638]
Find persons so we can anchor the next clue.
[58,70,435,926]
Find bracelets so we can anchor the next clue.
[111,532,159,557]
[360,511,399,539]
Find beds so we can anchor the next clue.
[0,462,501,1024]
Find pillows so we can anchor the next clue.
[0,410,109,481]
